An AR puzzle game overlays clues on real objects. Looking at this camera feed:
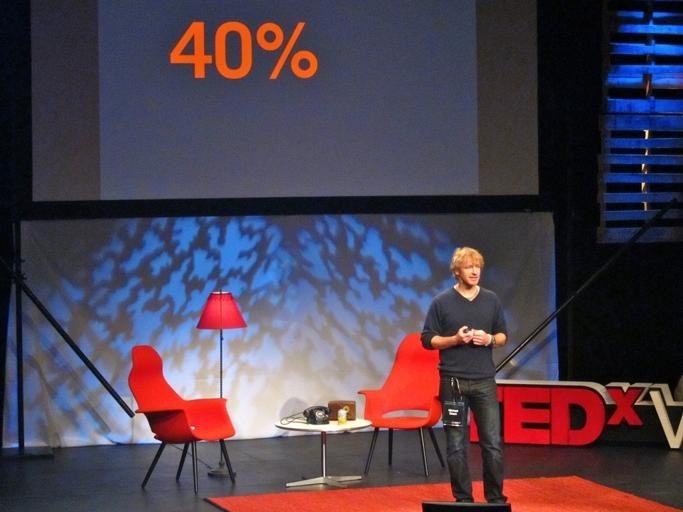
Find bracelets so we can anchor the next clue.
[484,334,493,348]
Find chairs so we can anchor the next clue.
[128,344,236,493]
[357,332,446,480]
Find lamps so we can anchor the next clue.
[197,288,248,479]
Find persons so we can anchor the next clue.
[421,242,509,504]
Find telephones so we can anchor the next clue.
[303,406,330,424]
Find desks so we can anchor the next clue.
[275,415,373,490]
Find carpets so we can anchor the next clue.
[203,472,683,512]
